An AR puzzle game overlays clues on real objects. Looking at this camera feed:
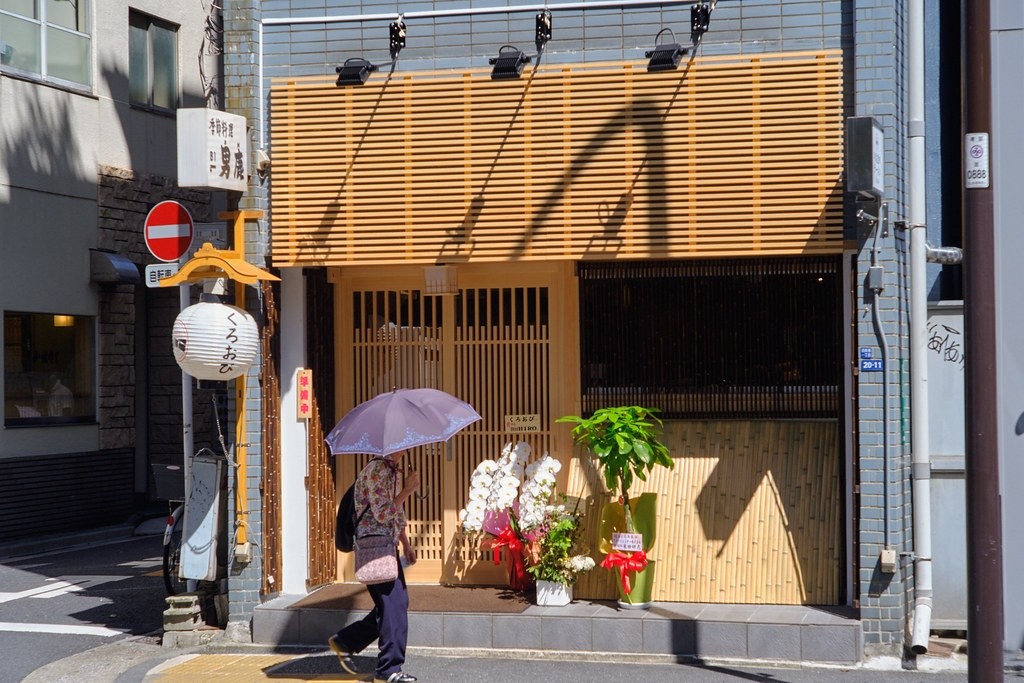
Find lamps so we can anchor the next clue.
[169,269,259,380]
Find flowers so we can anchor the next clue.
[455,439,598,585]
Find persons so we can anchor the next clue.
[327,448,418,682]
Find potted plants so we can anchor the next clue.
[554,405,678,609]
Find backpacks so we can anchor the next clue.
[334,457,385,553]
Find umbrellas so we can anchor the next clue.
[322,388,482,501]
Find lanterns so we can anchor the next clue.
[172,301,258,382]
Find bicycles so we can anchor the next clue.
[162,496,200,595]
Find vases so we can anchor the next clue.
[534,576,573,607]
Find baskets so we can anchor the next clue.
[151,463,185,500]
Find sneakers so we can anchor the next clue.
[373,671,417,683]
[328,633,358,676]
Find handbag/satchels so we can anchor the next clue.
[355,535,399,584]
